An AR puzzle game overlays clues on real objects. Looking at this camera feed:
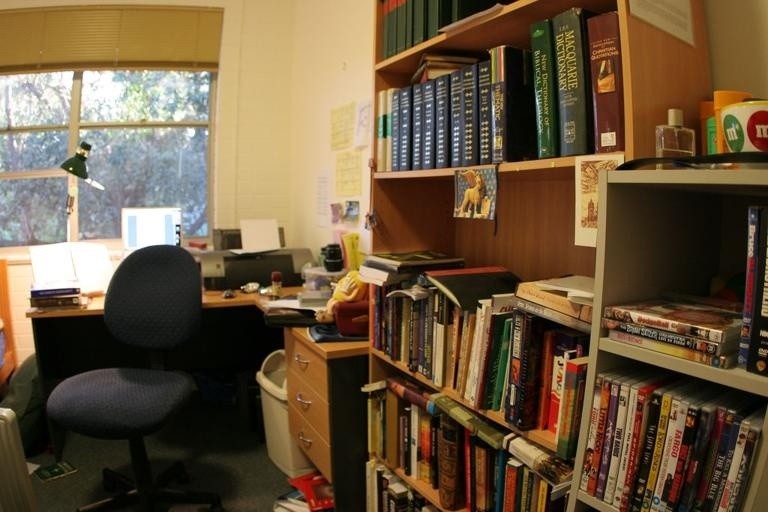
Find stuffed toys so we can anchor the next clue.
[315,269,368,323]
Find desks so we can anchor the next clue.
[25,280,369,512]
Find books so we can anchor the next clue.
[34,459,78,482]
[386,87,401,172]
[399,86,412,171]
[413,0,429,47]
[491,46,537,163]
[580,370,767,512]
[299,291,331,307]
[436,74,451,169]
[406,61,469,86]
[388,0,397,57]
[530,19,559,159]
[361,352,572,512]
[427,0,451,40]
[587,11,625,154]
[610,329,739,369]
[747,207,768,378]
[451,70,461,168]
[479,60,493,165]
[383,0,388,59]
[461,63,478,167]
[376,89,386,171]
[422,79,435,170]
[600,317,740,356]
[273,469,335,512]
[406,0,413,50]
[451,0,496,24]
[418,53,480,67]
[30,279,88,311]
[397,0,405,54]
[552,8,601,156]
[604,298,743,343]
[738,206,759,369]
[30,242,113,297]
[392,91,400,170]
[413,83,422,170]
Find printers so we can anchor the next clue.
[200,226,316,292]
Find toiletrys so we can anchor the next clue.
[656,108,697,171]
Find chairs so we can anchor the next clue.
[44,245,222,511]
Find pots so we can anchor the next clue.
[615,151,768,170]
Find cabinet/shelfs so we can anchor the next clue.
[0,258,19,394]
[366,0,632,512]
[567,164,768,512]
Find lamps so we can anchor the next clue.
[0,140,92,180]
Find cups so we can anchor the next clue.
[720,98,768,153]
